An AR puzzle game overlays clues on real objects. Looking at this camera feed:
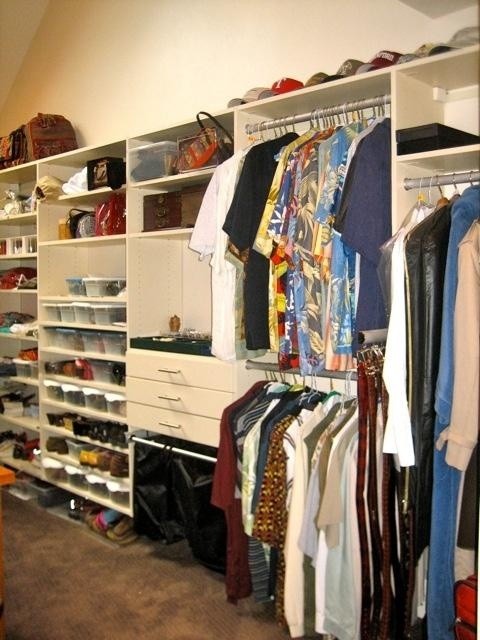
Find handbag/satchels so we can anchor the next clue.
[173,112,234,174]
[0,113,77,170]
[142,182,208,232]
[59,193,126,240]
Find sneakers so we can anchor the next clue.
[0,431,40,469]
[69,496,138,544]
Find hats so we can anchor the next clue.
[228,27,480,108]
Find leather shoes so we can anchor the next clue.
[47,412,129,477]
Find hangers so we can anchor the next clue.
[415,171,475,204]
[241,91,390,146]
[260,361,358,397]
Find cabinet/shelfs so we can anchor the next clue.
[0,44,480,517]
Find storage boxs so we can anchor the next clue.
[43,276,126,417]
[7,440,129,508]
[11,355,38,378]
[127,140,179,184]
